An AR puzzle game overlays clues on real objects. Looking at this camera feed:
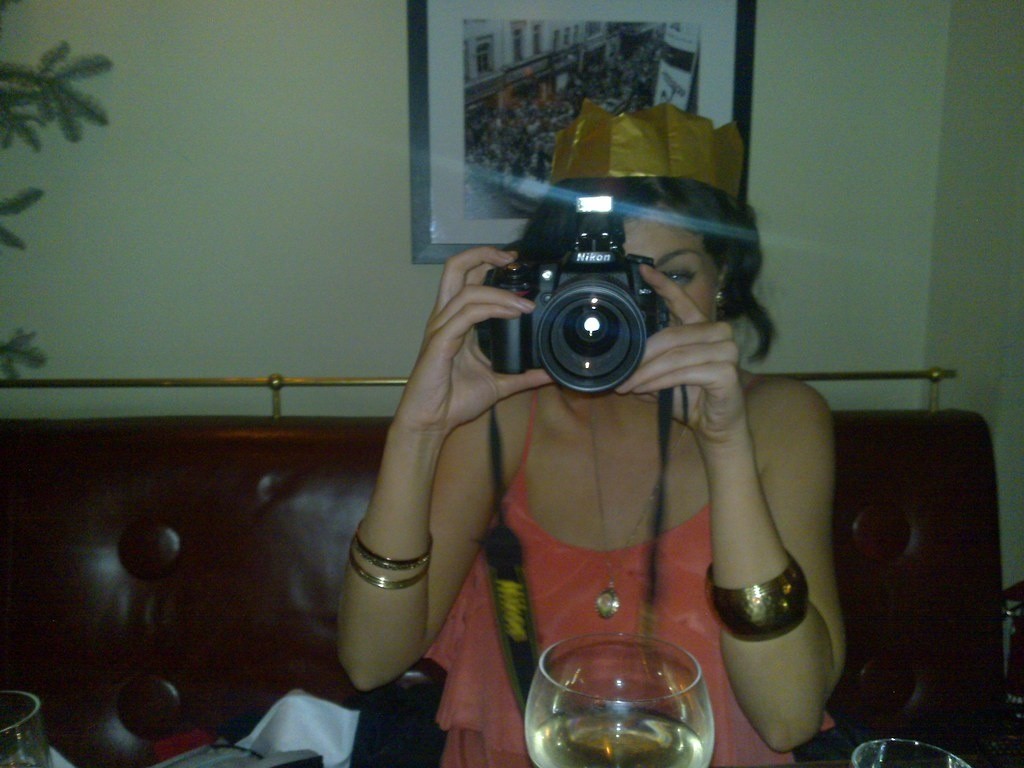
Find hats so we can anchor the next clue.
[549,100,745,200]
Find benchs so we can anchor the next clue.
[0,366,1018,768]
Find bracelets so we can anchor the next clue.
[704,548,808,642]
[349,518,432,592]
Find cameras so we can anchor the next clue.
[493,194,670,393]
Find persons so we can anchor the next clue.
[336,100,847,768]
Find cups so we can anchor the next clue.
[848,738,971,768]
[525,632,715,768]
[0,690,51,768]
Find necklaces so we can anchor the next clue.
[586,398,688,618]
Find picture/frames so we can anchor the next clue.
[407,0,756,268]
